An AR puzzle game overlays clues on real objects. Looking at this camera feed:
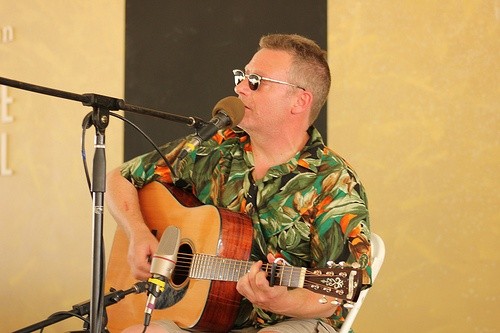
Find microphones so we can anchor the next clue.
[178,95,245,162]
[143,226,181,326]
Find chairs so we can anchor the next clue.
[336,233,385,331]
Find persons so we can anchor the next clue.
[101,34,372,333]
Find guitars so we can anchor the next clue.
[99,179,362,333]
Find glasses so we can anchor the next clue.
[233,69,306,92]
